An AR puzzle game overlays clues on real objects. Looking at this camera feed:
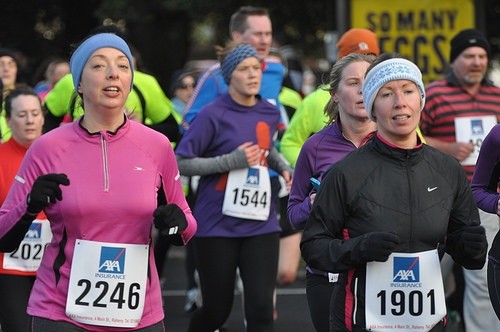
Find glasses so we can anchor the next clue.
[179,80,197,90]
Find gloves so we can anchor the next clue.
[27,173,70,215]
[152,204,187,236]
[461,225,487,261]
[358,230,401,262]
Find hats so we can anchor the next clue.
[336,28,379,63]
[448,28,491,65]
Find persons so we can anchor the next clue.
[287,52,390,332]
[0,0,298,332]
[300,53,486,332]
[468,121,500,332]
[278,27,500,205]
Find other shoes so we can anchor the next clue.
[184,288,202,313]
